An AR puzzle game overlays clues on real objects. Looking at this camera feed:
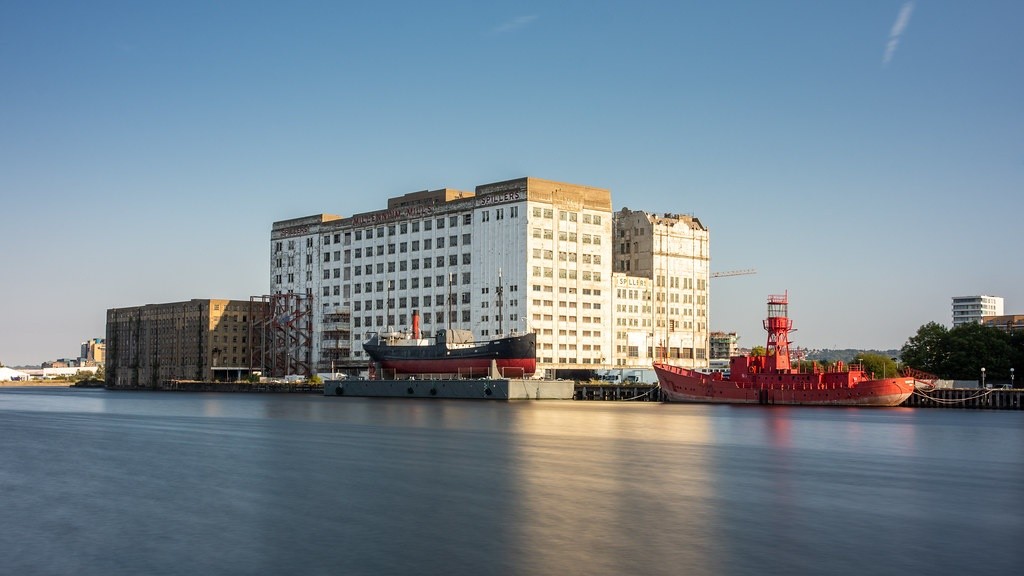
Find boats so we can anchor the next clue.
[361,310,540,378]
[652,290,915,406]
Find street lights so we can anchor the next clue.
[981,367,985,387]
[1010,368,1015,389]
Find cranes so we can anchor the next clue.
[709,269,756,278]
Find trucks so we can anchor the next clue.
[316,373,349,383]
[284,374,308,383]
[604,368,647,383]
[626,370,660,386]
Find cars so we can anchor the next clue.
[993,384,1012,389]
